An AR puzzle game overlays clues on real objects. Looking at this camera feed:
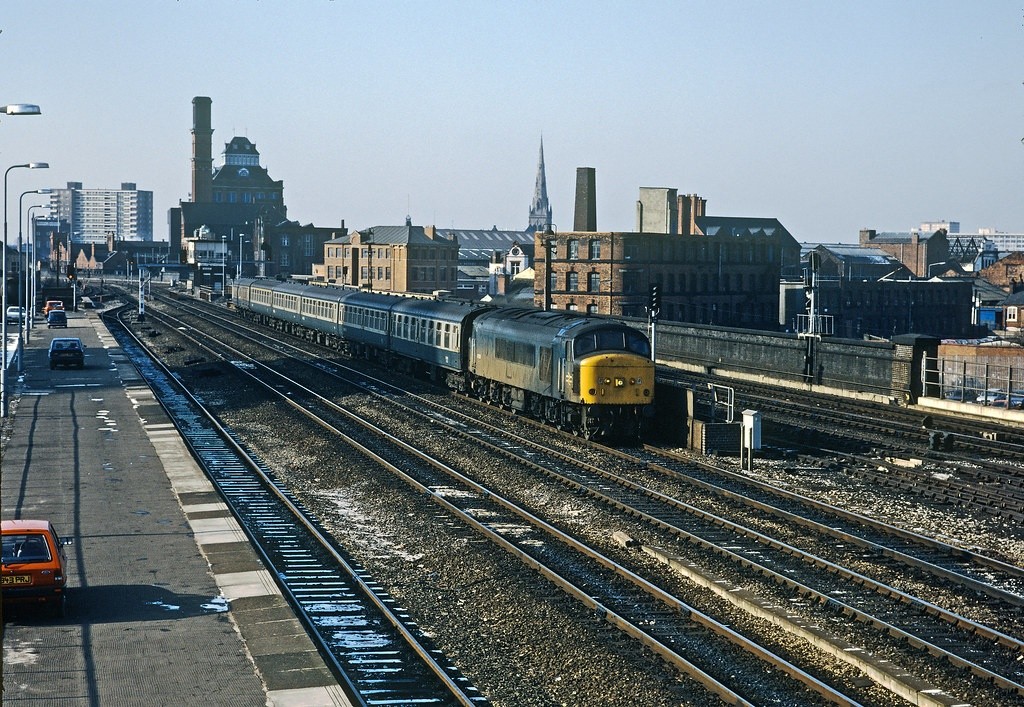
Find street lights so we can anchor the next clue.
[1,162,49,418]
[17,190,52,372]
[239,233,244,278]
[222,236,226,296]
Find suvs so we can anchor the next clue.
[0,520,73,617]
[43,301,67,329]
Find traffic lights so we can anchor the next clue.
[649,282,662,309]
[67,265,74,281]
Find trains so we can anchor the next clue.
[232,277,655,441]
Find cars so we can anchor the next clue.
[7,306,26,325]
[48,337,87,370]
